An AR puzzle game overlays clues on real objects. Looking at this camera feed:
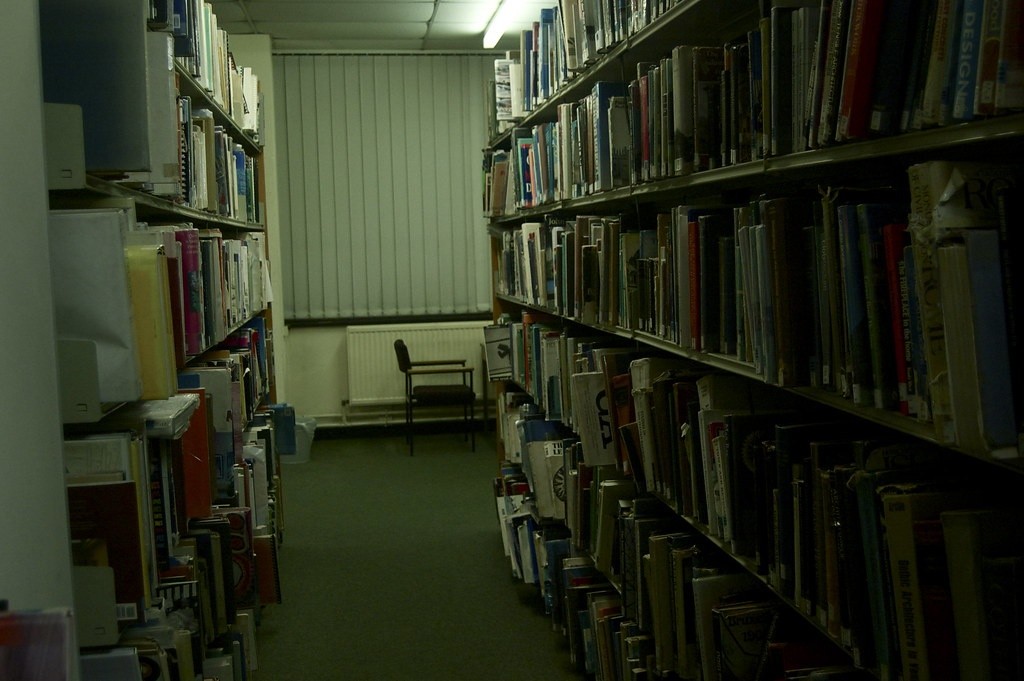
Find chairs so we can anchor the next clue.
[394,337,478,456]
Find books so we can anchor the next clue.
[482,0,1024,681]
[0,0,295,681]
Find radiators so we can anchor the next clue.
[346,321,495,403]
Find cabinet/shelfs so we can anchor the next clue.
[0,0,284,681]
[486,0,1024,681]
